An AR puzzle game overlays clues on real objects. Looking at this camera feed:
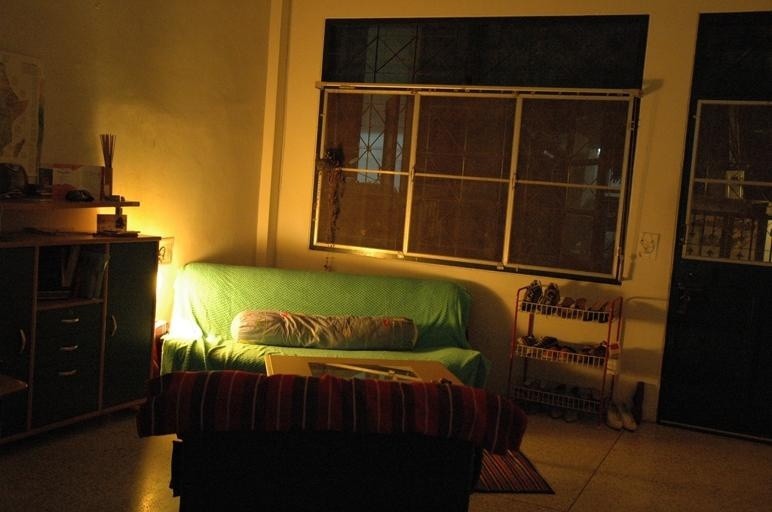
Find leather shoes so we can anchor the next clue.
[521,280,614,323]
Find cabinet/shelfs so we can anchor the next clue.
[0,246,37,444]
[509,276,623,427]
[104,239,160,413]
[32,241,104,429]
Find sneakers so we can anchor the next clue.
[513,377,600,422]
[517,335,606,365]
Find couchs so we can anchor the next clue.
[135,371,531,512]
[159,260,493,387]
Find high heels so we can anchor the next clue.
[605,399,638,431]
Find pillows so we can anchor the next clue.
[230,308,419,352]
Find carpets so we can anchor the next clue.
[477,447,555,494]
